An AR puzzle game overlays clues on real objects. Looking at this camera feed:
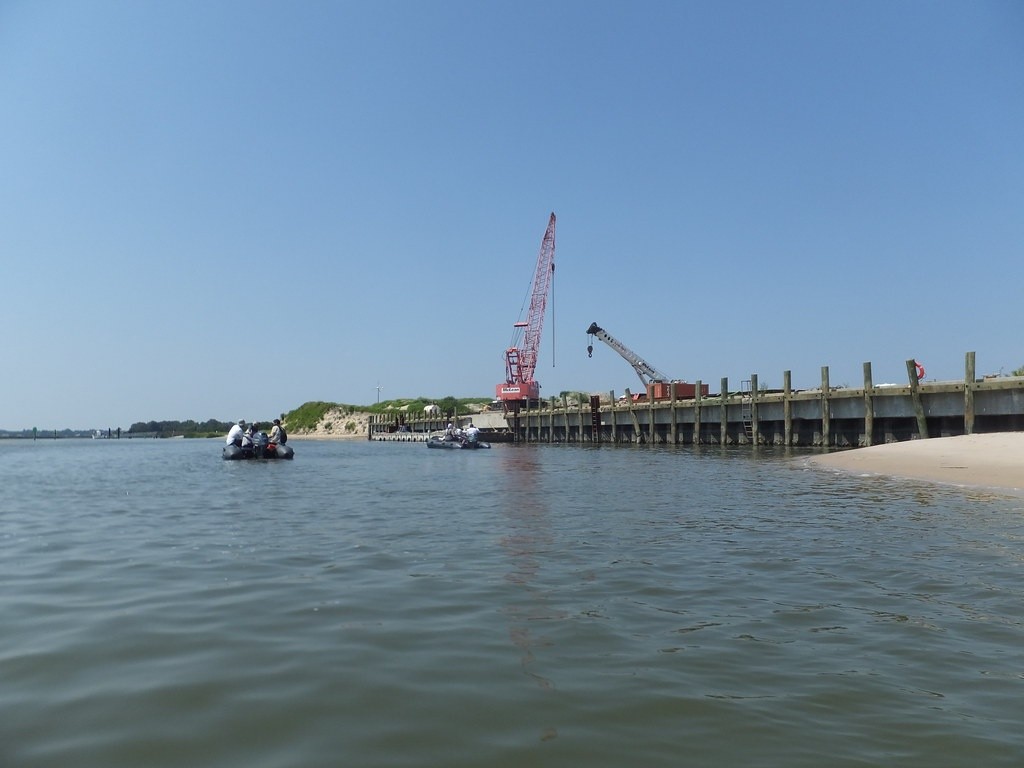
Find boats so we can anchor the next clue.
[427,436,492,450]
[222,431,295,462]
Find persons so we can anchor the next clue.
[396,415,478,447]
[226,418,287,457]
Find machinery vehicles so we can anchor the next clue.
[586,321,709,402]
[494,211,557,411]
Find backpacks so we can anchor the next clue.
[278,426,287,443]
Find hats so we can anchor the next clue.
[252,421,260,427]
[239,419,245,427]
